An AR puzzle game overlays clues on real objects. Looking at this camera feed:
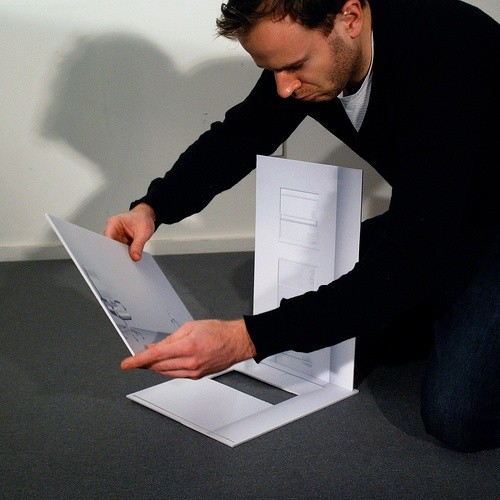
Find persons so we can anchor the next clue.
[105,0,500,456]
